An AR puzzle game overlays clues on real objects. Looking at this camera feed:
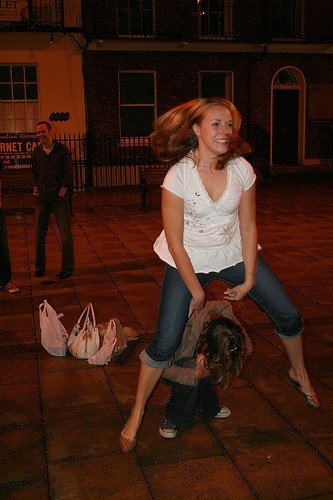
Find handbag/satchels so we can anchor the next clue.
[39,299,69,356]
[87,319,117,365]
[96,318,140,357]
[67,302,99,360]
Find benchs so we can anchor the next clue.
[0,169,74,216]
[139,167,168,211]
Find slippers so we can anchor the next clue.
[120,434,137,453]
[287,377,321,409]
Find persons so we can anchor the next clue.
[120,97,319,453]
[31,121,74,279]
[0,199,20,293]
[158,300,253,438]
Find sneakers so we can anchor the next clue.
[214,404,231,419]
[159,417,177,438]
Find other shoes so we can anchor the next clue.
[56,268,73,279]
[34,270,45,277]
[5,282,20,293]
